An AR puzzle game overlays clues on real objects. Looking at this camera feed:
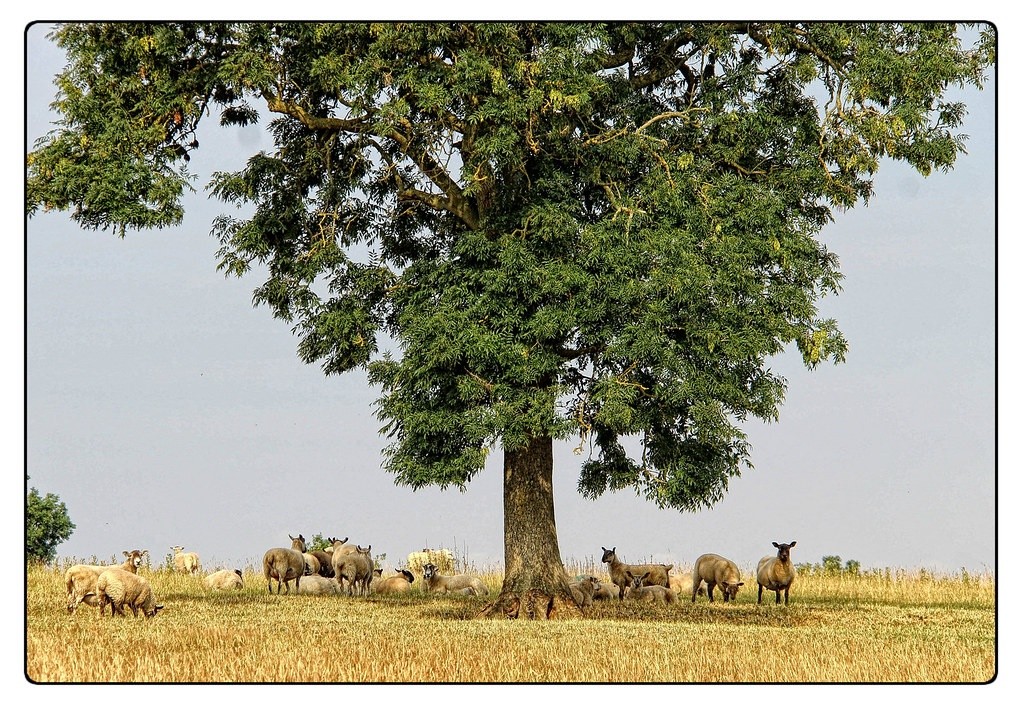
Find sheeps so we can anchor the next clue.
[170,545,200,574]
[601,546,706,606]
[691,553,744,605]
[421,563,489,598]
[203,569,244,593]
[569,577,620,609]
[262,533,415,596]
[757,541,797,605]
[65,549,164,620]
